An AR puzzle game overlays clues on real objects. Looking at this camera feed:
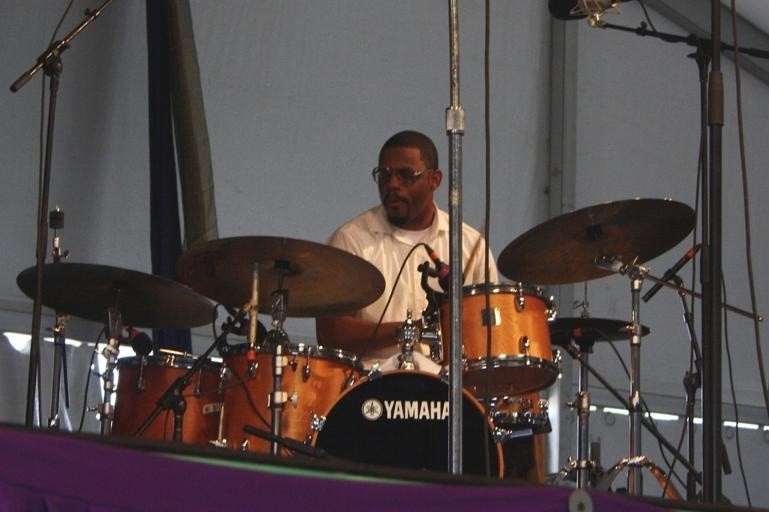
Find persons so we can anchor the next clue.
[317,130,502,373]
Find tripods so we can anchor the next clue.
[553,339,615,494]
[590,259,688,506]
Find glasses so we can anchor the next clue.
[372,167,429,183]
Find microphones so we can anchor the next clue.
[427,244,462,289]
[636,244,703,301]
[547,1,613,20]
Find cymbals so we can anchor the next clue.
[548,318,651,343]
[498,198,697,285]
[17,263,217,329]
[175,236,385,317]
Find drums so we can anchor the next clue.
[312,370,504,480]
[436,285,560,398]
[111,356,225,445]
[222,343,366,458]
[479,392,551,438]
